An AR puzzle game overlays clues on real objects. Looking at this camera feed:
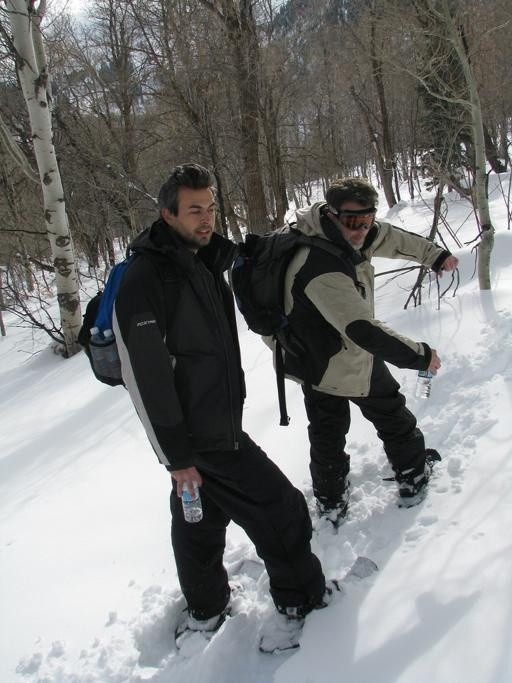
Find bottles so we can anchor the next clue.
[414,367,433,399]
[88,326,109,380]
[103,328,122,380]
[180,478,204,525]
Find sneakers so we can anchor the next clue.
[269,578,342,632]
[392,449,441,506]
[314,480,350,528]
[189,581,244,633]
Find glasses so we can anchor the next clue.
[328,205,377,230]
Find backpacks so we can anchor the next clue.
[78,251,142,386]
[232,220,359,337]
